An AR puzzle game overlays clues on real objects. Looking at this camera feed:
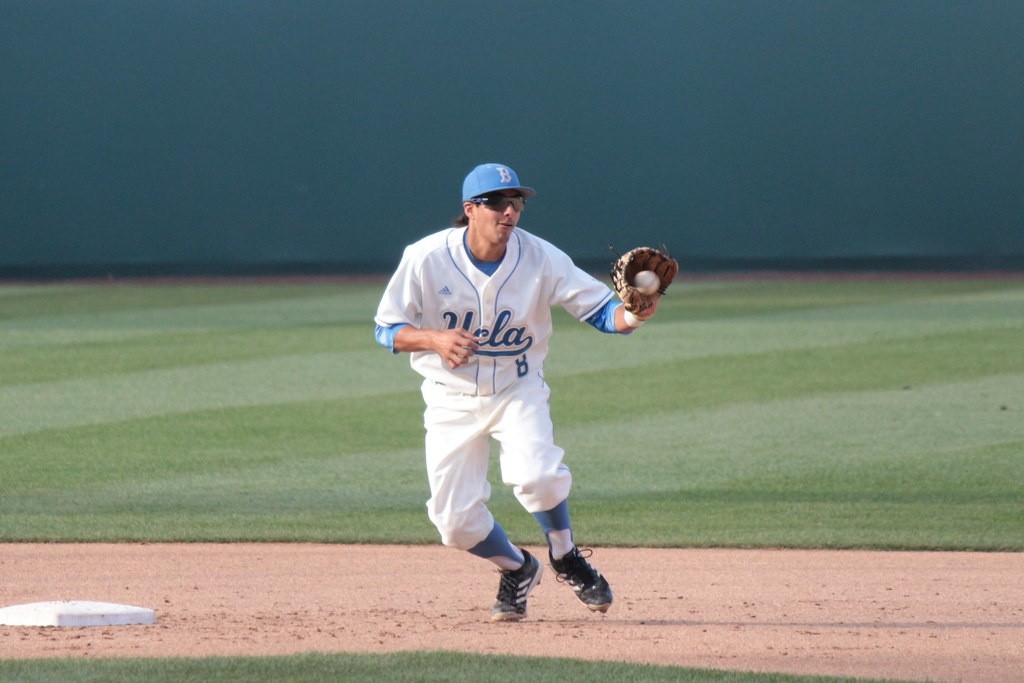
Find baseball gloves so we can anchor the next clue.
[608,242,679,321]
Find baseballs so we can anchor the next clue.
[634,270,661,295]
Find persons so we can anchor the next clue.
[375,163,662,621]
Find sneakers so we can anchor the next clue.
[492,546,542,623]
[551,546,614,611]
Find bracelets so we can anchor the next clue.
[623,310,644,328]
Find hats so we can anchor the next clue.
[462,163,536,202]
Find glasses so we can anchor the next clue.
[468,197,530,212]
